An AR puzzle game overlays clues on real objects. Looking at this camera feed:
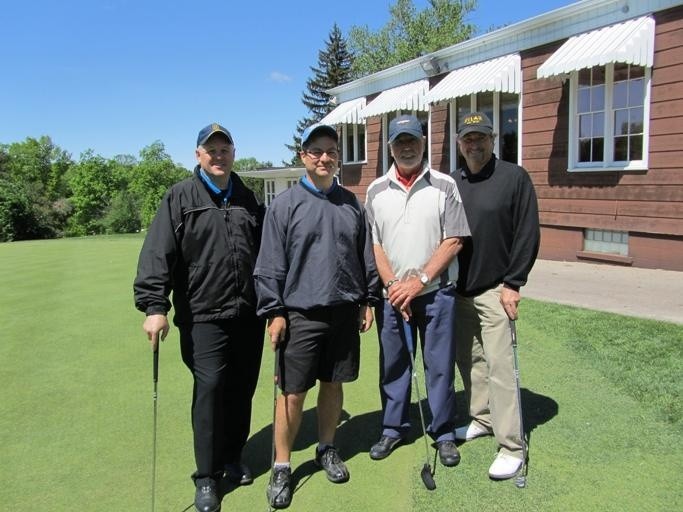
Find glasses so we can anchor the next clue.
[305,151,339,159]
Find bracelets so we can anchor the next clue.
[384,279,399,288]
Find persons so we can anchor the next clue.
[361,114,475,467]
[131,122,268,511]
[445,112,541,481]
[252,121,382,510]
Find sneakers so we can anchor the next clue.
[371,434,415,459]
[315,447,349,483]
[455,420,492,440]
[267,464,292,508]
[438,440,460,466]
[489,451,523,479]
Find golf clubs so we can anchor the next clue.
[401,316,435,490]
[507,318,528,488]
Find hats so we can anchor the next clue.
[197,124,234,148]
[457,112,493,138]
[301,124,339,151]
[388,115,422,144]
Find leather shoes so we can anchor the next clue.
[195,485,221,512]
[224,461,253,484]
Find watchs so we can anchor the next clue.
[419,272,430,285]
